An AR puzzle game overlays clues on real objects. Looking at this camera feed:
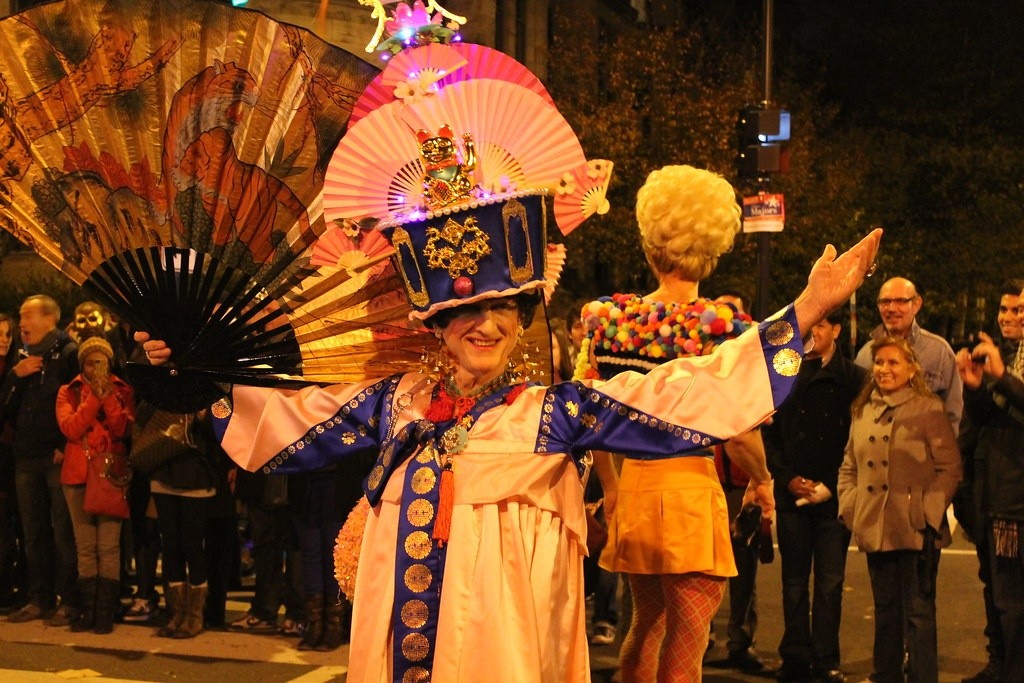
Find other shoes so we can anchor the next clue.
[816,665,848,683]
[960,661,999,683]
[730,644,775,676]
[775,660,815,683]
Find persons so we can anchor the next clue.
[133,228,883,682]
[550,302,633,642]
[0,294,79,627]
[837,277,1024,683]
[74,301,106,340]
[131,390,216,639]
[56,336,135,634]
[572,164,776,683]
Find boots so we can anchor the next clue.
[93,577,119,634]
[315,597,344,652]
[158,582,186,637]
[173,581,208,639]
[71,578,97,632]
[297,591,323,650]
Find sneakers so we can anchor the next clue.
[226,613,276,633]
[50,604,82,626]
[278,618,305,635]
[7,602,45,622]
[123,597,159,621]
[591,620,617,644]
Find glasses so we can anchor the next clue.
[880,296,916,306]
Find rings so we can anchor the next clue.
[145,350,150,358]
[801,478,805,483]
[865,262,876,279]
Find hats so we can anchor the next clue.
[78,336,115,370]
[381,194,547,321]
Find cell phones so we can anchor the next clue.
[969,338,987,362]
[95,358,107,372]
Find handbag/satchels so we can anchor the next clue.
[84,452,134,518]
[130,410,198,471]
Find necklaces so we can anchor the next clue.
[432,370,513,549]
